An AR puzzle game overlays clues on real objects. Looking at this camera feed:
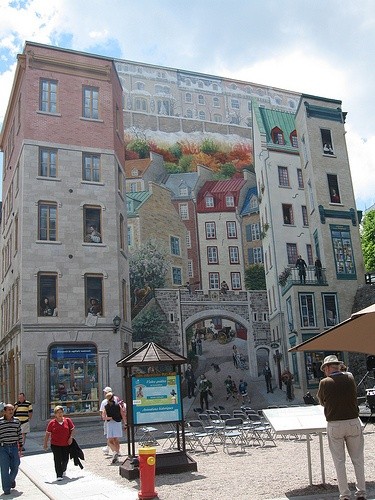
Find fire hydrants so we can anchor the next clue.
[137,448,159,500]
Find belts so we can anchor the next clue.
[4,441,18,447]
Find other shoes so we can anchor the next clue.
[103,446,110,455]
[112,451,121,464]
[57,477,64,481]
[63,471,67,477]
[118,450,122,457]
[19,446,27,451]
[11,480,17,488]
[4,489,11,495]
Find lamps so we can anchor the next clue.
[112,314,121,333]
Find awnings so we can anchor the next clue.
[288,304,375,355]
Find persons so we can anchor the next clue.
[317,355,367,500]
[102,392,127,463]
[56,383,67,401]
[100,387,126,435]
[0,404,23,495]
[14,393,33,451]
[44,406,75,481]
[72,382,80,391]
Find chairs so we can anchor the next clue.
[160,404,319,456]
[136,426,160,448]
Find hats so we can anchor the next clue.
[319,355,343,372]
[103,387,113,393]
